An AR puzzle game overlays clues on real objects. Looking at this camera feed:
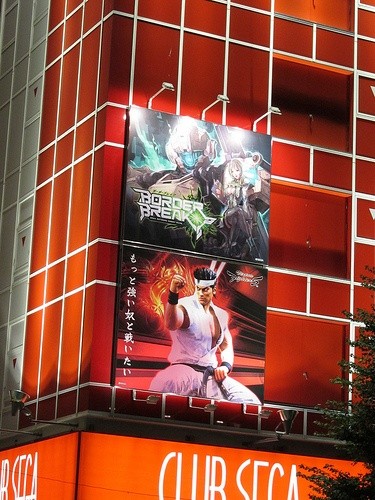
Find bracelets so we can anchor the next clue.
[220,361,232,374]
[167,290,179,305]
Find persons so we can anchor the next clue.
[148,267,263,405]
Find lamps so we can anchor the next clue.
[129,390,159,405]
[200,94,230,121]
[252,106,282,133]
[273,407,298,440]
[8,389,81,428]
[241,403,272,419]
[188,398,218,412]
[147,82,175,108]
